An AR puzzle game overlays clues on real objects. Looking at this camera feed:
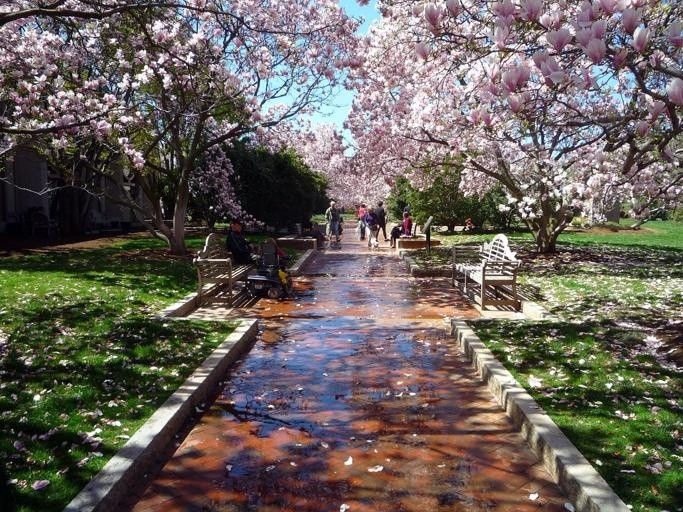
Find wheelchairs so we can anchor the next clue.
[247,238,294,298]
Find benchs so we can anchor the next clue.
[294,222,314,238]
[399,222,417,238]
[448,232,523,312]
[192,232,261,310]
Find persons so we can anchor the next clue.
[324,201,341,242]
[265,238,285,256]
[356,201,412,251]
[227,220,260,265]
